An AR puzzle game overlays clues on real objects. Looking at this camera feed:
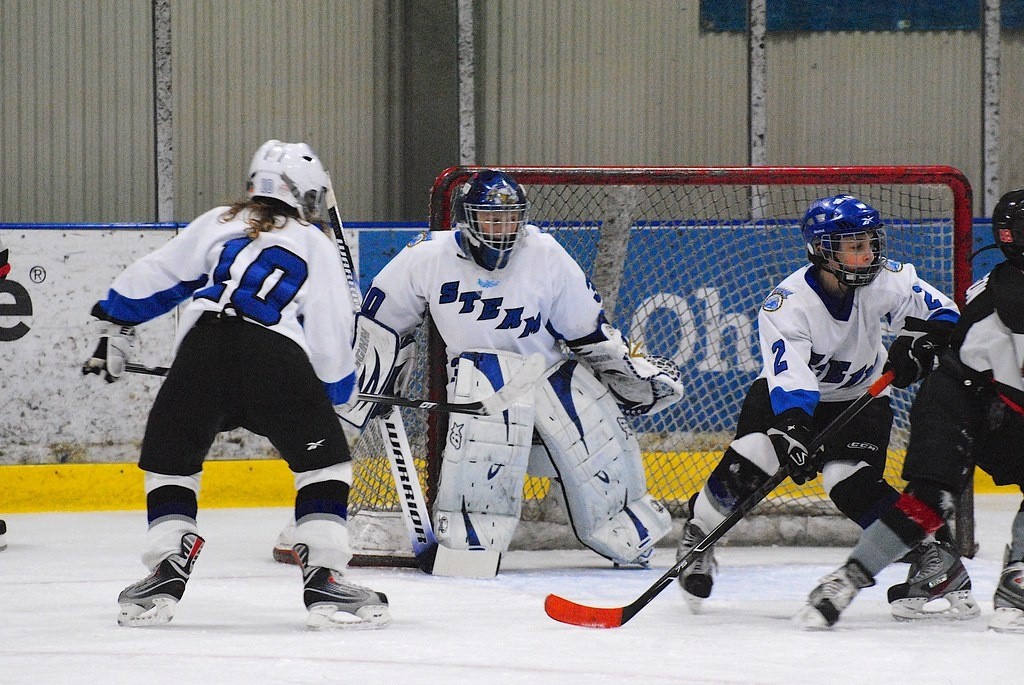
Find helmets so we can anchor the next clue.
[800,193,887,285]
[991,189,1024,272]
[247,140,330,221]
[457,170,526,271]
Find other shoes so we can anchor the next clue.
[0,519,13,551]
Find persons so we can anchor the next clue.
[362,169,685,579]
[84,139,390,630]
[677,189,1024,634]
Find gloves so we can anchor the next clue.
[881,317,937,389]
[764,407,824,485]
[79,319,139,383]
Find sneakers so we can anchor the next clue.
[294,543,393,629]
[989,544,1024,631]
[676,506,717,599]
[795,558,875,628]
[886,540,981,624]
[112,533,206,626]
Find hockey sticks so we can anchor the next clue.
[319,170,501,579]
[87,350,546,416]
[543,369,894,629]
